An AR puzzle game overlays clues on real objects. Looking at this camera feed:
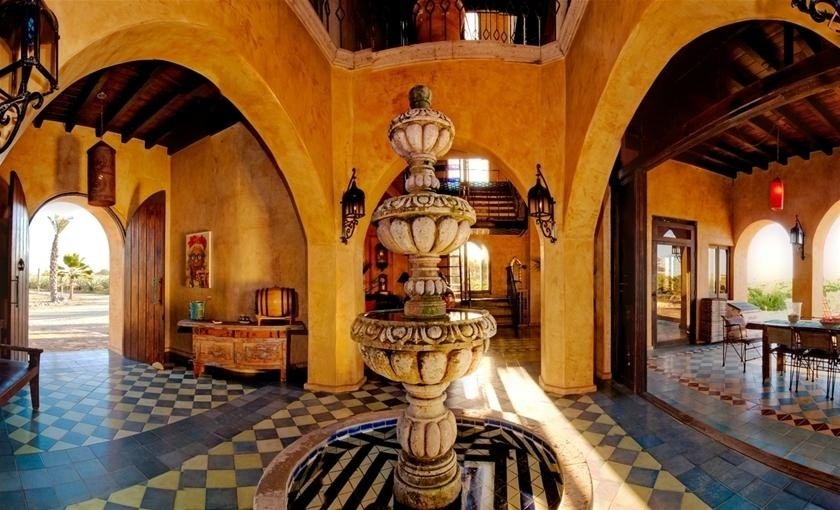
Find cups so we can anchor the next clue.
[785,302,803,325]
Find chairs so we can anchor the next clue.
[0,344,44,409]
[721,313,840,401]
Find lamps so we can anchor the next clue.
[527,163,557,243]
[672,244,682,263]
[87,92,115,206]
[375,241,388,271]
[340,168,365,245]
[0,0,61,157]
[789,215,805,260]
[768,126,785,212]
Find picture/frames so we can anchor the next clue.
[185,231,211,289]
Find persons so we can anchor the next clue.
[187,235,208,288]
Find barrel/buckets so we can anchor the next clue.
[254,286,296,317]
